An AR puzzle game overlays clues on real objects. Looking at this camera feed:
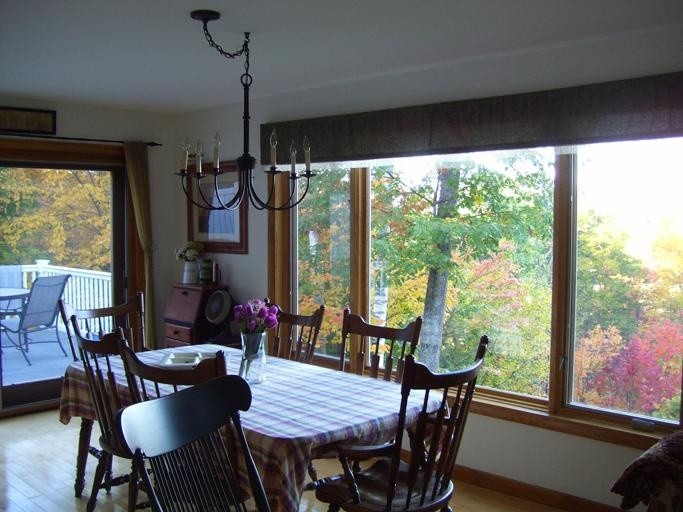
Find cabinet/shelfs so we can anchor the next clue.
[163,284,230,348]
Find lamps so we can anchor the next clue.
[175,9,317,211]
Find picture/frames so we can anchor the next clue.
[186,160,249,254]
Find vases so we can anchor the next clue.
[238,331,267,384]
[183,260,198,284]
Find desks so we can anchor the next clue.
[0,287,30,353]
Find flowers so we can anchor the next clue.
[232,298,279,334]
[172,241,203,263]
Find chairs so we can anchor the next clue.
[70,315,138,512]
[112,375,272,511]
[0,274,71,366]
[336,307,422,470]
[59,291,144,361]
[263,297,327,364]
[118,338,227,403]
[315,335,490,512]
